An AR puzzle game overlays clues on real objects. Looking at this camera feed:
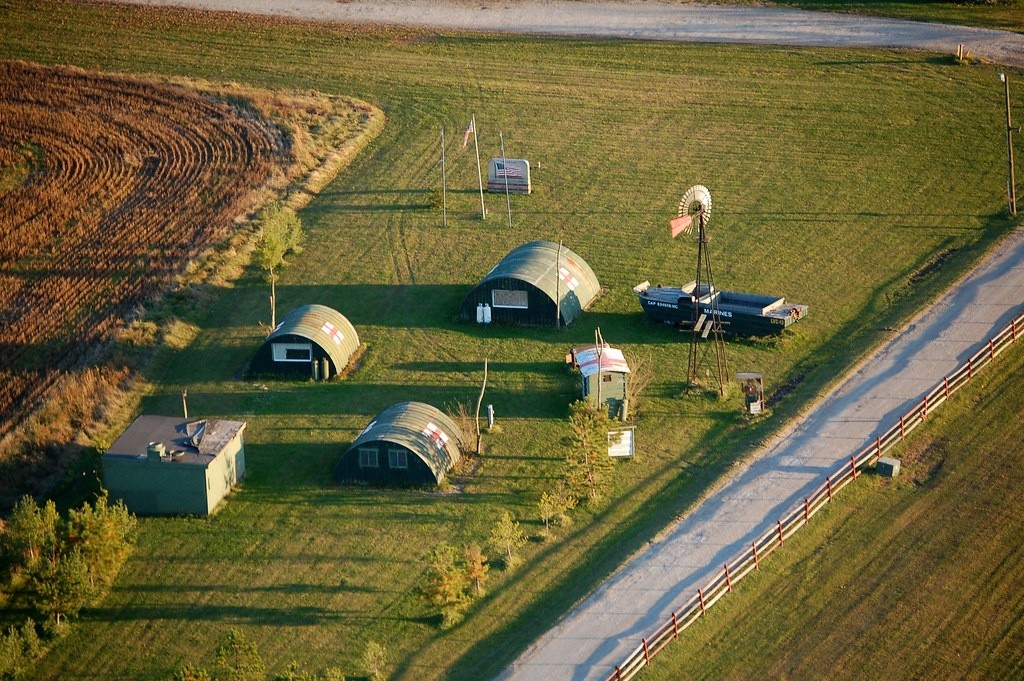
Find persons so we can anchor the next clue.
[741,379,763,412]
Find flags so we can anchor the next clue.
[463,119,474,147]
